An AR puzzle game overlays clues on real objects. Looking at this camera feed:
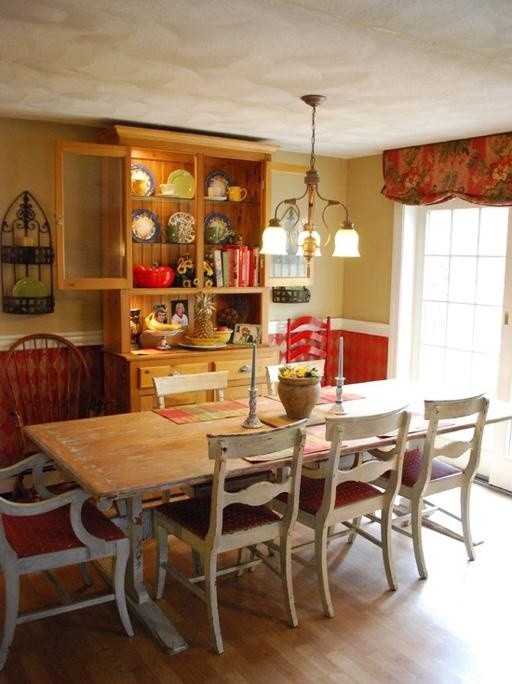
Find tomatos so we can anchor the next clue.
[133,263,175,288]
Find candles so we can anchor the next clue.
[251,344,257,391]
[337,337,344,377]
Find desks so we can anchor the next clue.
[22,372,506,655]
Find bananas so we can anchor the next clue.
[144,311,181,331]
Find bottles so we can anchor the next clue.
[130,306,144,349]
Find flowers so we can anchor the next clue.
[176,248,213,288]
[278,366,317,377]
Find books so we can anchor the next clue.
[209,245,263,288]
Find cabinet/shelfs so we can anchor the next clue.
[51,124,280,503]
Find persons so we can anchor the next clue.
[155,309,167,323]
[173,302,188,325]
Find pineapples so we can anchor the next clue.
[193,319,213,339]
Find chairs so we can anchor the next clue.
[248,404,411,620]
[0,332,116,512]
[152,418,311,654]
[265,358,376,522]
[285,318,331,389]
[152,370,276,577]
[329,392,490,579]
[0,453,134,673]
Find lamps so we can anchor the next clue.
[258,94,362,279]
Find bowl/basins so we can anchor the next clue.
[185,335,223,345]
[213,328,233,344]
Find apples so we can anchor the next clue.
[217,326,229,331]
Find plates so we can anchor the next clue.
[130,165,232,243]
[178,341,227,350]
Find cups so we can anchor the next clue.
[228,185,248,201]
[20,235,34,246]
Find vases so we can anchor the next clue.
[278,377,321,420]
